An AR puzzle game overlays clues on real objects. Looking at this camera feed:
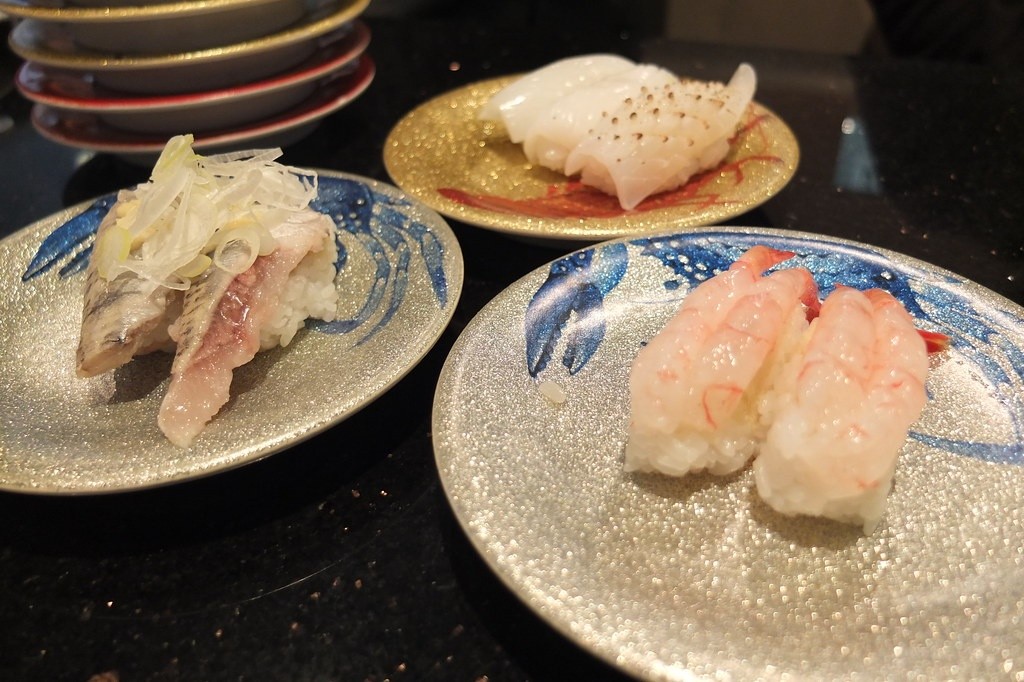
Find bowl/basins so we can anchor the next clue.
[0,0,376,154]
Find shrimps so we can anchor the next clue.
[752,282,953,537]
[623,245,822,479]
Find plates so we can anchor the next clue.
[0,166,466,498]
[432,225,1024,682]
[382,74,802,241]
